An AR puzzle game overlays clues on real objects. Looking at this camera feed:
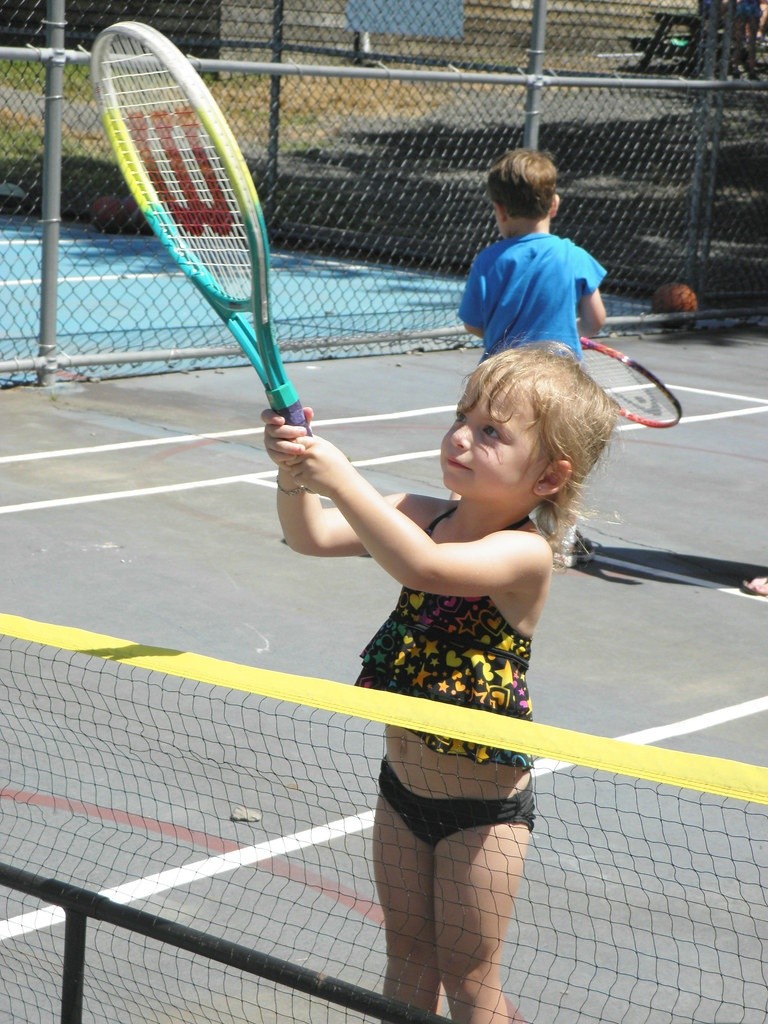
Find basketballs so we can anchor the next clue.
[651,282,698,329]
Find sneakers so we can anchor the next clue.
[552,529,594,567]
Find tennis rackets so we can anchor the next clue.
[576,335,682,428]
[89,20,318,495]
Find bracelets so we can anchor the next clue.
[276,475,309,496]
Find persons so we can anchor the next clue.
[456,148,609,406]
[698,0,762,81]
[260,341,619,1024]
